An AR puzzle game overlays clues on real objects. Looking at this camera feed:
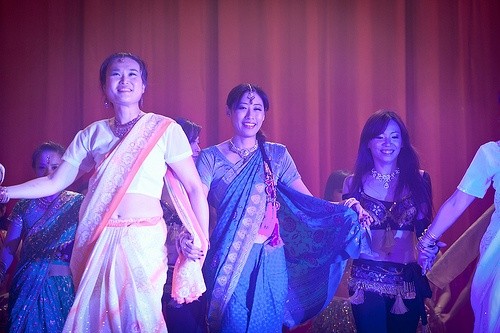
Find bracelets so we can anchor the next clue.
[437,303,444,309]
[416,225,438,252]
[178,230,191,245]
[0,186,10,203]
[343,197,360,208]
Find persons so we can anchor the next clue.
[417,138,500,333]
[175,83,373,333]
[0,51,211,332]
[338,108,435,332]
[314,170,358,333]
[0,142,83,332]
[160,115,202,332]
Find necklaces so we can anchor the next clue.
[228,139,260,162]
[371,168,401,190]
[38,197,53,208]
[110,114,142,136]
